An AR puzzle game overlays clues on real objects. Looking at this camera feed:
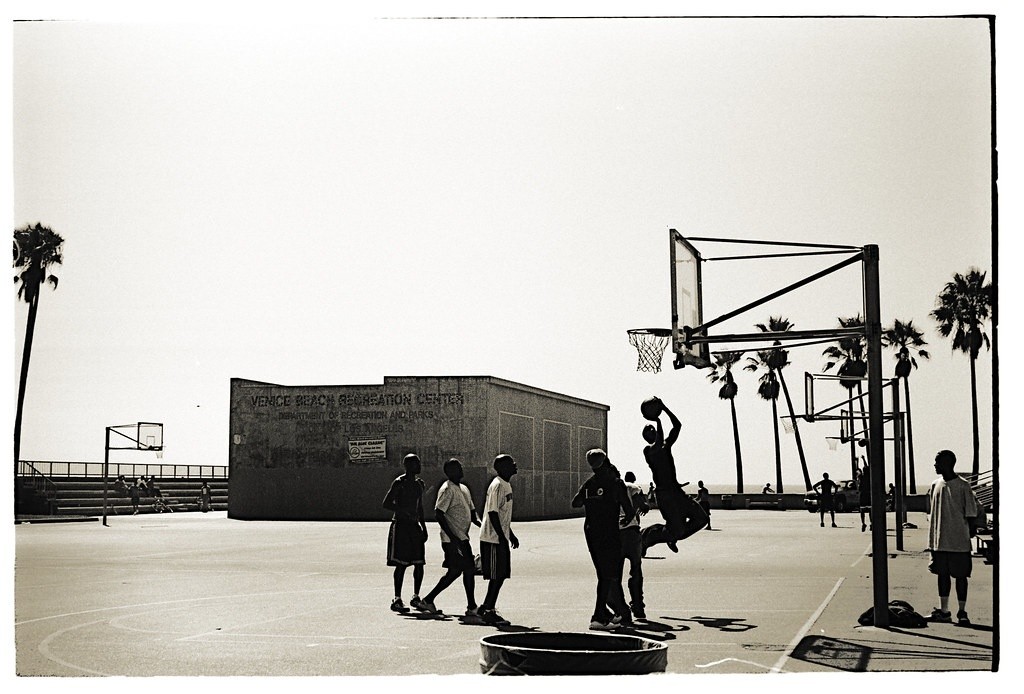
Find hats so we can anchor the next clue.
[586,449,606,468]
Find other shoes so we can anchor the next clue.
[667,540,679,554]
[704,526,711,530]
[390,599,410,613]
[612,615,625,623]
[589,620,621,630]
[632,605,646,618]
[477,604,505,624]
[821,523,824,527]
[862,524,866,532]
[410,596,421,608]
[832,523,837,527]
[640,542,647,558]
[418,598,441,614]
[465,605,478,616]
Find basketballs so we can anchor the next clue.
[858,438,867,447]
[640,397,663,417]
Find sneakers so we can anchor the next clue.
[957,611,970,625]
[923,607,952,622]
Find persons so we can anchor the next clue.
[696,481,711,530]
[201,481,212,510]
[926,450,987,625]
[418,458,481,616]
[858,455,873,532]
[763,483,775,503]
[382,454,426,612]
[641,396,708,554]
[888,484,895,512]
[813,473,837,527]
[115,475,162,515]
[572,448,645,629]
[478,454,519,626]
[647,482,656,507]
